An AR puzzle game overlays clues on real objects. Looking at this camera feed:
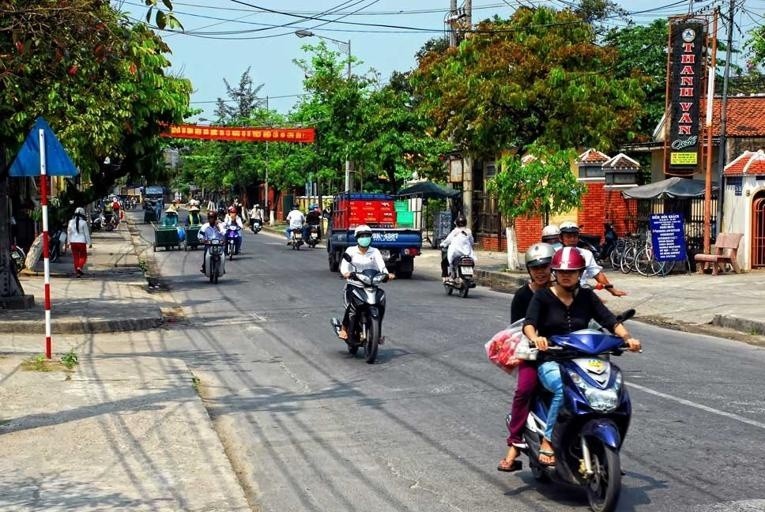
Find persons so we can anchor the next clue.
[197,210,226,274]
[439,214,475,286]
[497,241,559,469]
[550,219,629,298]
[65,206,94,278]
[520,245,641,461]
[89,191,225,226]
[222,205,244,253]
[284,201,304,246]
[302,203,322,239]
[246,203,265,229]
[540,224,561,244]
[338,225,397,339]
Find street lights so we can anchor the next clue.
[295,27,356,196]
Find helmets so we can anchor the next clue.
[208,205,238,217]
[456,216,466,227]
[354,224,372,239]
[292,203,319,210]
[524,222,587,271]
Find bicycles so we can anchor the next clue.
[610,231,678,277]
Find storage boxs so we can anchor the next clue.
[332,199,414,228]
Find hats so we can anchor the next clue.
[188,205,201,212]
[164,204,177,215]
[74,208,86,216]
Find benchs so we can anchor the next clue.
[694,233,744,276]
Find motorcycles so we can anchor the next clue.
[289,226,303,248]
[333,252,396,365]
[555,218,618,260]
[516,323,630,511]
[305,223,322,249]
[91,197,139,231]
[441,238,478,299]
[187,196,266,282]
[48,230,66,263]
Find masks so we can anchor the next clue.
[357,237,372,248]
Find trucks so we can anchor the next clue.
[325,194,423,278]
[143,185,165,211]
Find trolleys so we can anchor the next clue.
[152,224,181,251]
[184,223,204,252]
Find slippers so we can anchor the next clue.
[539,450,557,466]
[497,458,522,472]
